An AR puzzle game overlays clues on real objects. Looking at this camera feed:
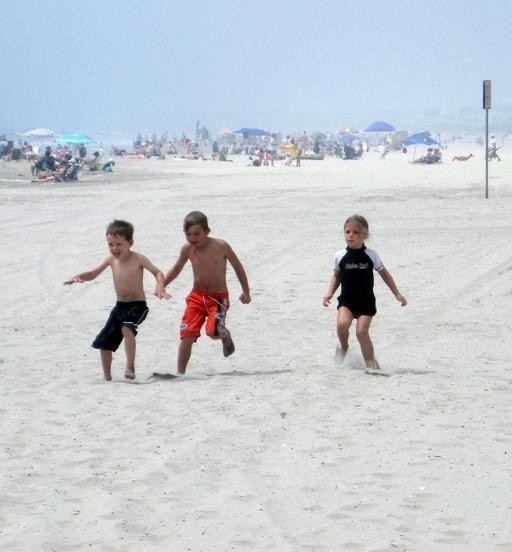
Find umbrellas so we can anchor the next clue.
[363,121,394,138]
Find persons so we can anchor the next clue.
[63,218,172,382]
[322,214,407,366]
[164,211,252,374]
[2,129,101,183]
[488,136,500,161]
[401,132,442,163]
[113,128,364,167]
[452,153,472,161]
